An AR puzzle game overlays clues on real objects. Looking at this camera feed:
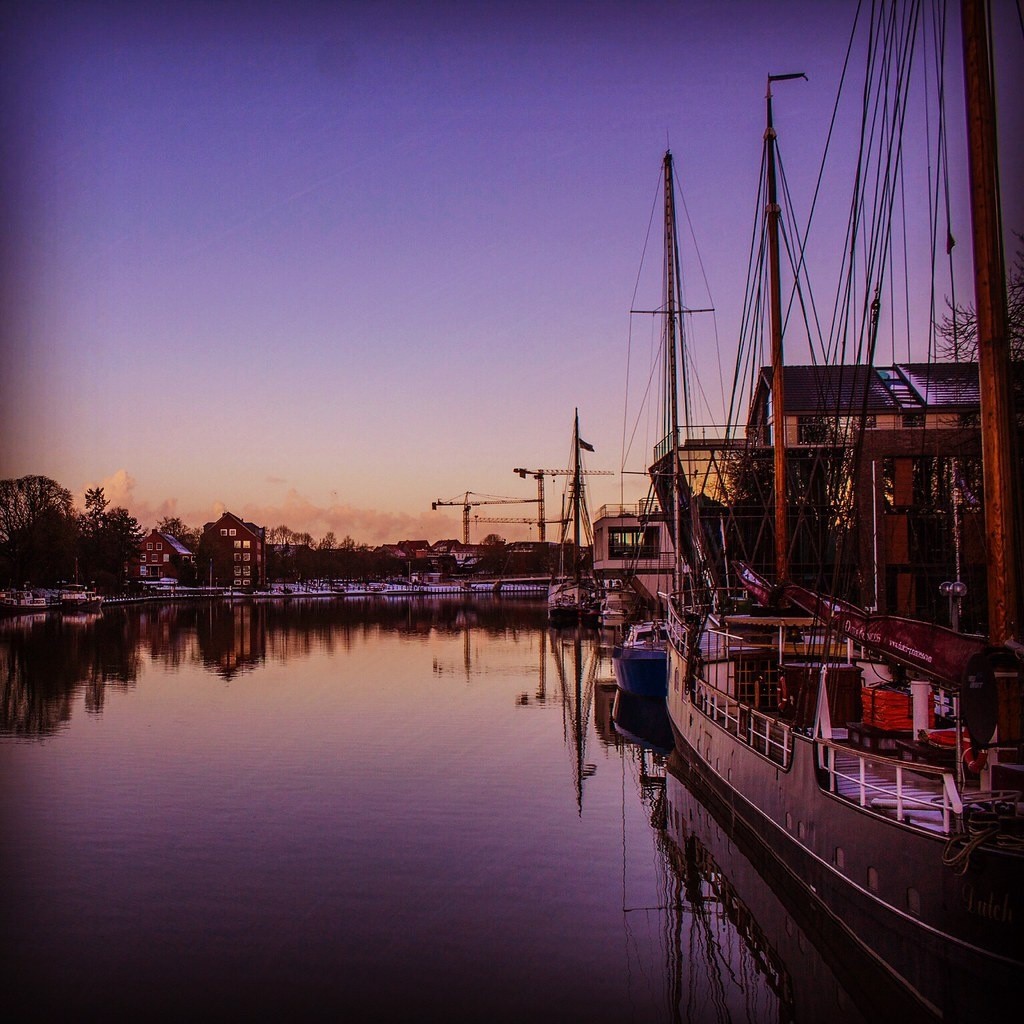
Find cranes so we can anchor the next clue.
[432,491,544,545]
[512,467,615,542]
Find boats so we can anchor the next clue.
[0,556,105,614]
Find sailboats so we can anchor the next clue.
[608,683,1023,1024]
[547,0,1024,997]
